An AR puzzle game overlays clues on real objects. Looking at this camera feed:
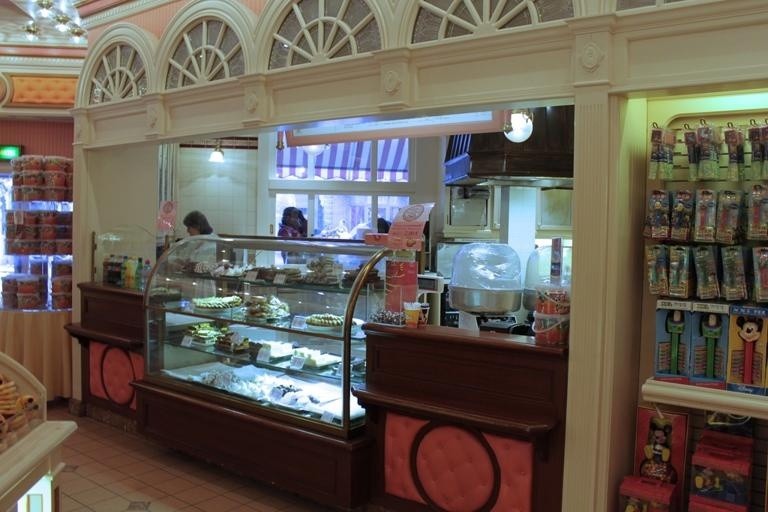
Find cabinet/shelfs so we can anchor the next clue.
[128,232,392,511]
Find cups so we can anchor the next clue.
[403,307,431,329]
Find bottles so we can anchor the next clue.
[101,253,151,293]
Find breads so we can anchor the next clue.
[181,254,357,369]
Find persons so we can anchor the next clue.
[275,207,306,263]
[168,207,218,310]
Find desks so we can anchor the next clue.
[1,308,72,403]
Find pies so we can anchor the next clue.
[0,380,19,414]
